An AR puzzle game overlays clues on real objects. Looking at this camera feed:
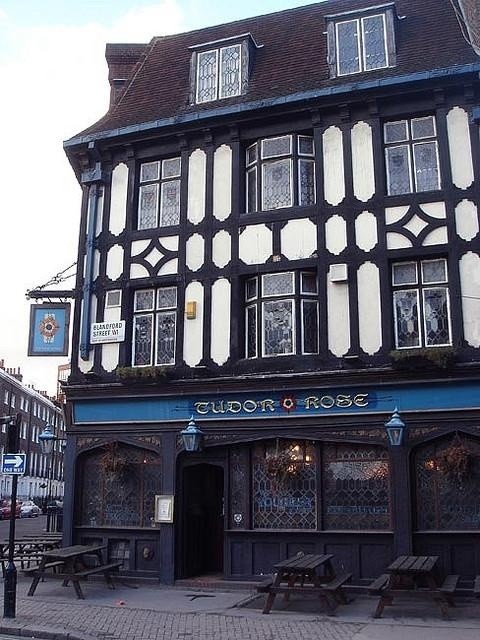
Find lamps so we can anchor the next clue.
[384,402,409,453]
[39,422,69,455]
[185,301,196,320]
[180,414,202,452]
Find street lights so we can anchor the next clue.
[39,482,48,513]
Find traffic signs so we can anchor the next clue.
[1,453,27,475]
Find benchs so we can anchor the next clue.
[253,547,480,620]
[1,530,123,602]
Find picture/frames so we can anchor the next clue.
[152,494,175,523]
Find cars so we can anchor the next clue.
[0,498,22,520]
[42,499,64,514]
[19,500,41,518]
